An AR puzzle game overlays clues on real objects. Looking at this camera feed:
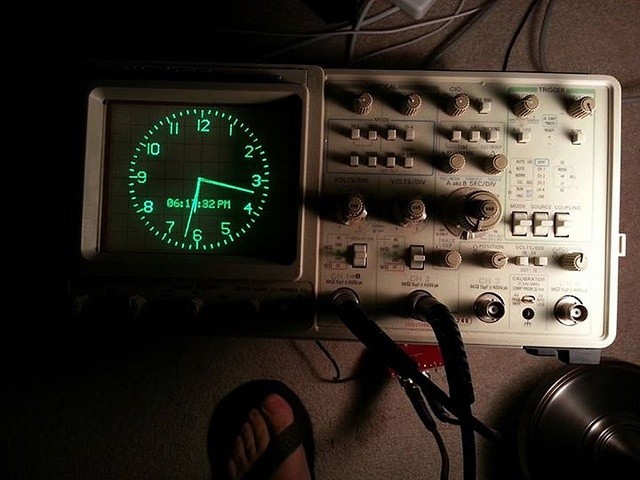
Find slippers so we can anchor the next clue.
[206,378,316,480]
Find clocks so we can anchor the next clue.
[101,98,301,265]
